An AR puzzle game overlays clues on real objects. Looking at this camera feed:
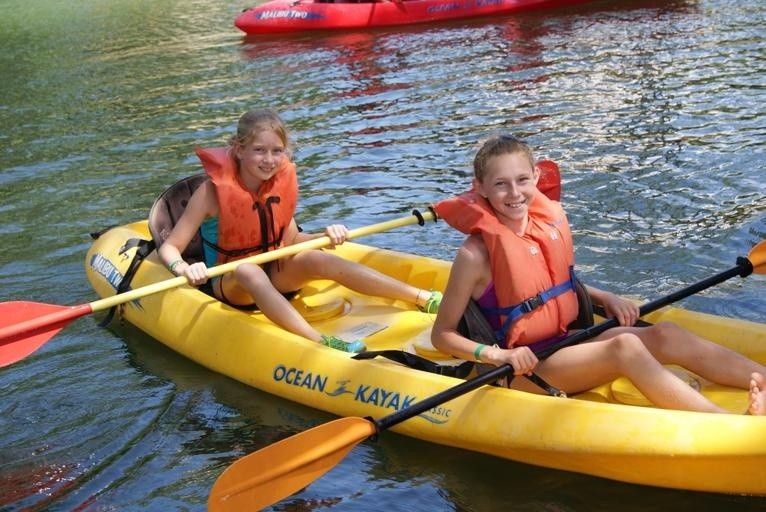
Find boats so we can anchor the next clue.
[85,218,766,496]
[233,1,587,34]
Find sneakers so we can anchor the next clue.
[320,334,365,353]
[416,290,443,314]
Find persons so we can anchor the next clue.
[158,109,444,353]
[430,135,766,416]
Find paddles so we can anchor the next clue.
[0,159,561,370]
[208,237,765,511]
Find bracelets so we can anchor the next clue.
[473,343,487,364]
[168,260,184,272]
